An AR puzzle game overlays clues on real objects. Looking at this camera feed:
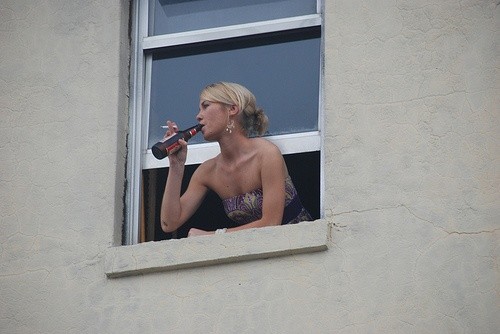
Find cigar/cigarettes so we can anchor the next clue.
[160,125,177,129]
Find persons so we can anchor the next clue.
[161,81,308,238]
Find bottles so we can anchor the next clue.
[151,125,205,160]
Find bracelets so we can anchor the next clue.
[215,228,227,235]
